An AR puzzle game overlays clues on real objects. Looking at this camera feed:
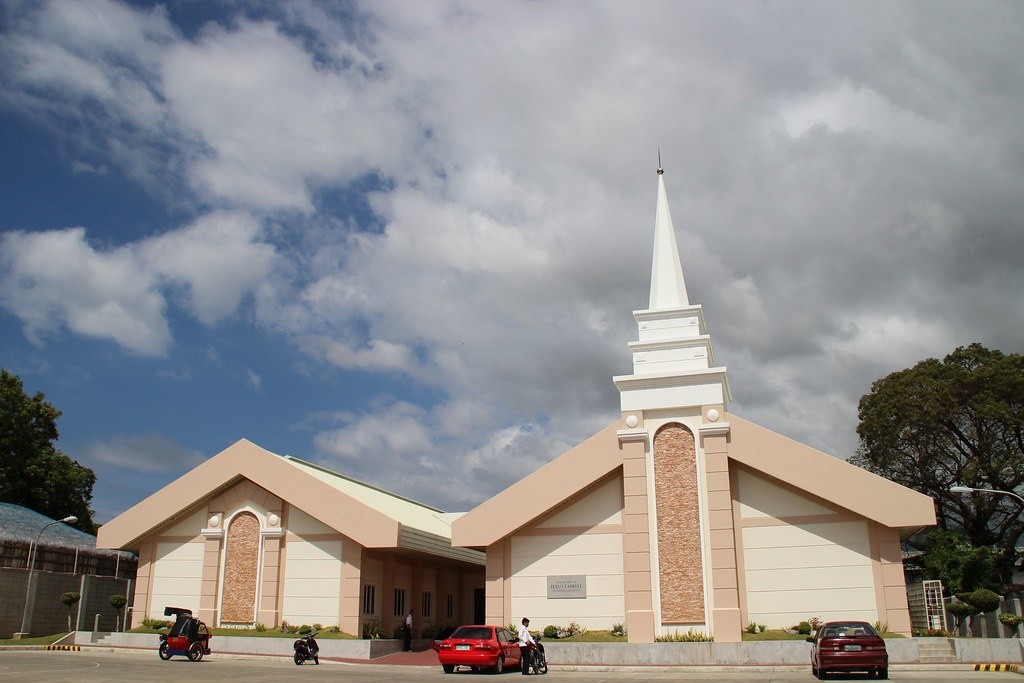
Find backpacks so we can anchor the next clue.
[401,615,408,631]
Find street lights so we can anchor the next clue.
[20,516,78,633]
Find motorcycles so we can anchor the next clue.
[159,615,211,662]
[527,635,548,674]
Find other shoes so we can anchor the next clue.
[405,649,412,652]
[522,671,531,675]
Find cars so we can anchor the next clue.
[433,624,523,674]
[806,621,888,680]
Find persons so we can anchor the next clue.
[404,609,415,651]
[519,618,539,675]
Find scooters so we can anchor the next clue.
[294,633,320,665]
